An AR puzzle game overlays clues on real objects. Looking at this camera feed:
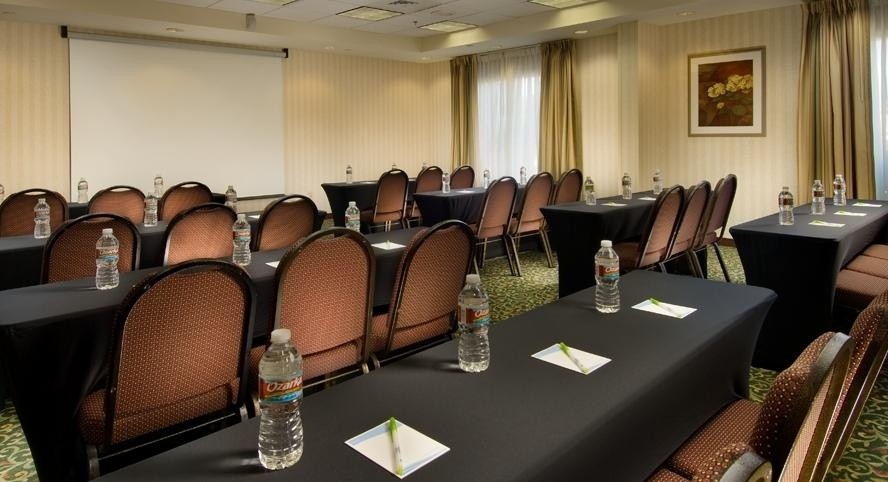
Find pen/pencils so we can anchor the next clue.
[650,297,681,316]
[387,239,390,248]
[390,417,404,475]
[560,341,588,375]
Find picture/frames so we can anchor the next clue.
[684,45,770,138]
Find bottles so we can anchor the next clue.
[653,169,663,194]
[777,186,795,226]
[622,172,632,200]
[811,180,827,215]
[345,202,360,231]
[77,176,89,204]
[832,173,848,206]
[593,240,621,313]
[458,273,492,374]
[520,166,527,185]
[584,176,597,206]
[392,162,397,170]
[154,176,164,199]
[346,164,354,184]
[94,228,120,290]
[224,185,237,212]
[483,168,489,190]
[256,329,304,469]
[232,213,252,267]
[144,192,158,227]
[422,163,427,169]
[33,198,52,239]
[441,170,451,194]
